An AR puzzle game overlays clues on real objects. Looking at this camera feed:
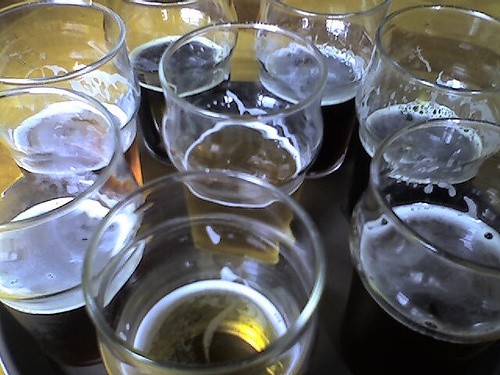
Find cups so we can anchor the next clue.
[0,0,499,375]
